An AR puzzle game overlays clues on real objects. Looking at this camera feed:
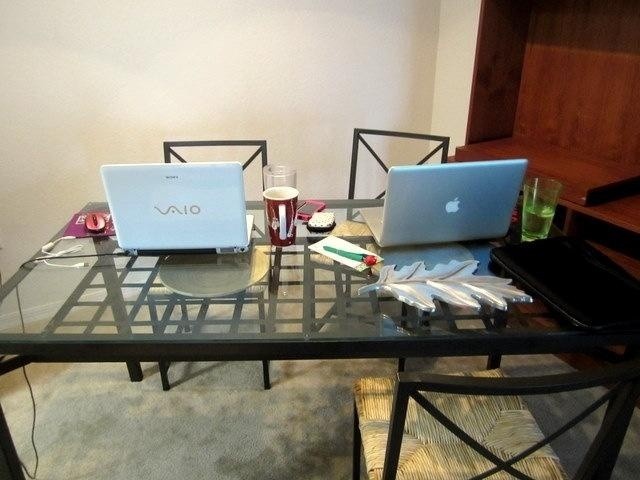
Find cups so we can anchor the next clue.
[521,177,562,241]
[263,162,298,247]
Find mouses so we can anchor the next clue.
[85,214,106,233]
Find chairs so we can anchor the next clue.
[352,357,640,480]
[300,128,450,336]
[145,141,271,390]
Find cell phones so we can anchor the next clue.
[296,200,326,221]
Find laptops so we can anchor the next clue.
[358,158,528,248]
[100,162,254,256]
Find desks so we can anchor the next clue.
[2,203,640,480]
[443,134,640,331]
